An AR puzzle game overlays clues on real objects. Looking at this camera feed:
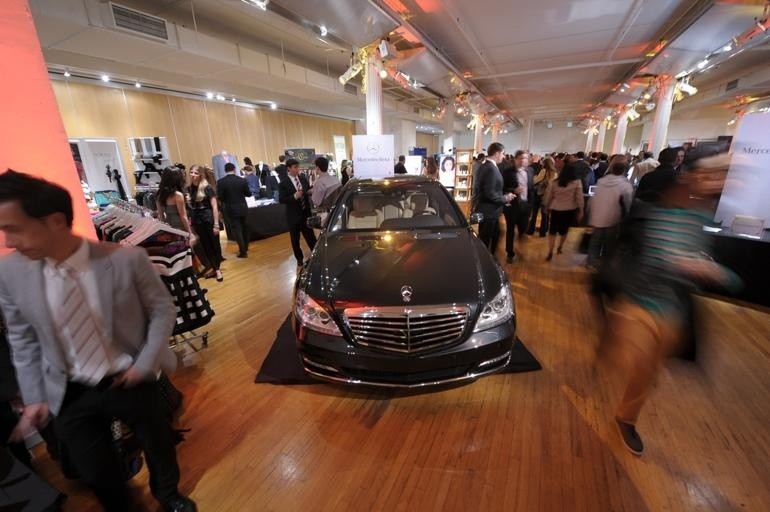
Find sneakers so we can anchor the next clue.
[615,415,645,456]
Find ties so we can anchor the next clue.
[295,177,306,210]
[59,267,111,388]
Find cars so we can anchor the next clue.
[291,175,517,395]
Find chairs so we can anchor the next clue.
[346,189,437,231]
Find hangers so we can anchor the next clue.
[90,196,192,255]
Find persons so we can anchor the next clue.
[216,163,252,258]
[420,157,440,181]
[0,169,200,512]
[278,159,318,267]
[306,156,343,233]
[439,156,456,186]
[471,141,741,455]
[394,155,407,174]
[240,154,287,201]
[154,163,227,282]
[342,159,354,187]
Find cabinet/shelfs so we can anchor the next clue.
[225,198,287,244]
[453,148,476,200]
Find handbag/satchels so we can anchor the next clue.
[537,179,548,195]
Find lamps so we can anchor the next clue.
[589,76,698,136]
[238,0,330,41]
[45,66,281,112]
